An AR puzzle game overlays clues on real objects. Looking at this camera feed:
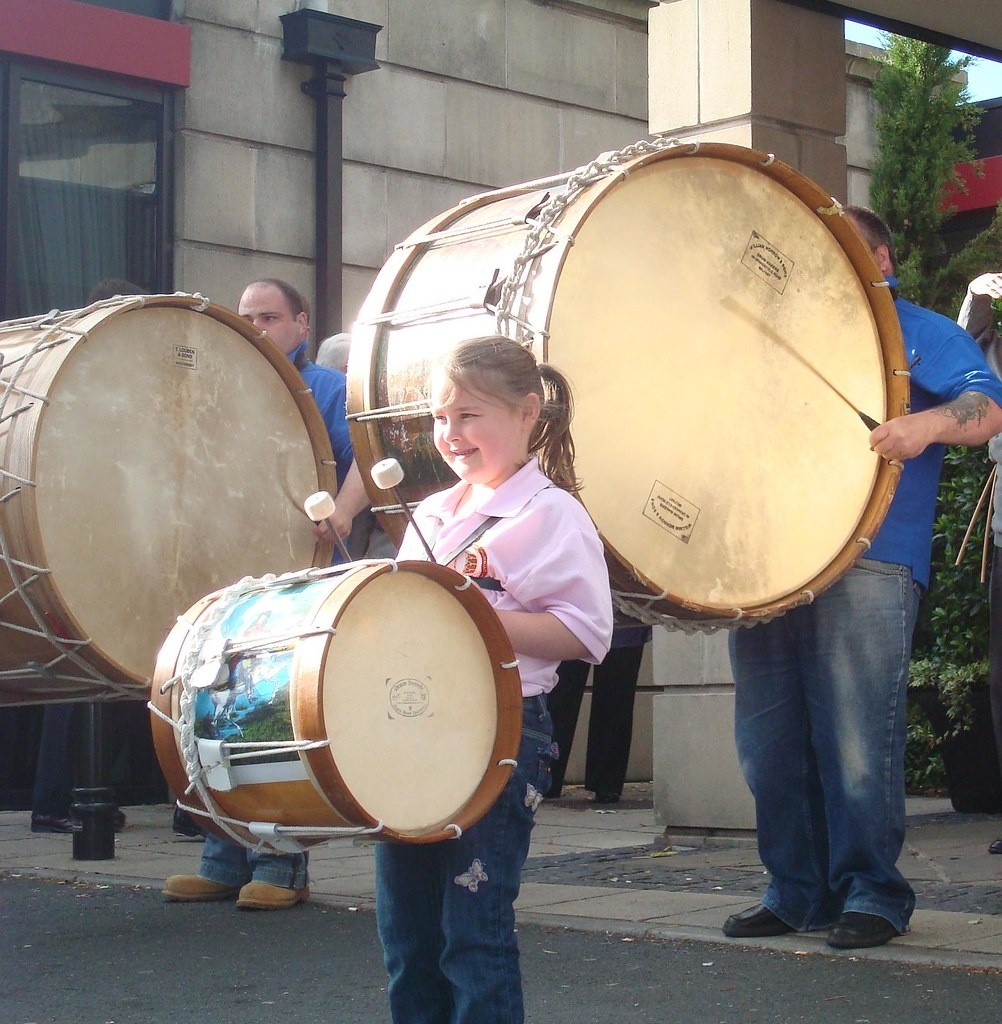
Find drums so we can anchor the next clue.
[150,557,524,847]
[0,289,339,700]
[343,141,910,626]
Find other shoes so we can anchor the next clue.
[595,793,620,803]
[161,875,239,901]
[236,881,310,909]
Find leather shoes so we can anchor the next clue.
[31,809,83,833]
[172,805,206,837]
[988,839,1002,854]
[722,904,793,937]
[827,912,897,948]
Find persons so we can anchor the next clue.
[30,281,149,834]
[163,276,372,912]
[316,332,356,373]
[542,553,654,804]
[372,337,614,1024]
[722,203,1002,948]
